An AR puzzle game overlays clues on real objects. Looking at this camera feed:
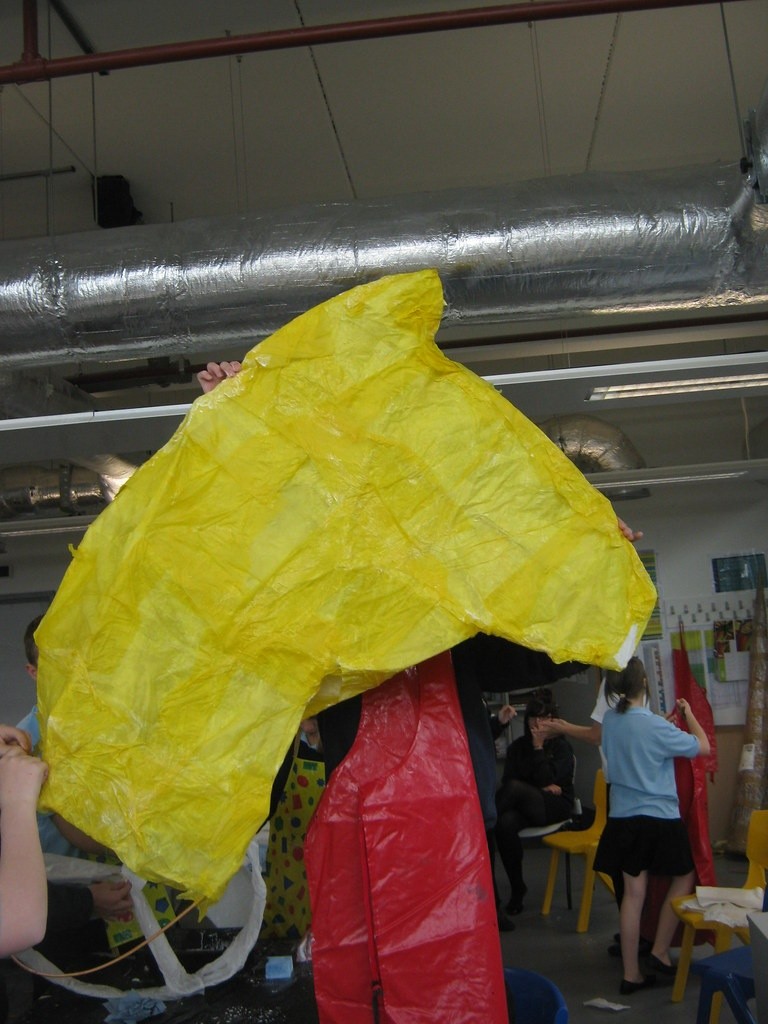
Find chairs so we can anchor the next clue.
[542,769,616,932]
[696,883,768,1024]
[518,755,583,909]
[670,810,768,1023]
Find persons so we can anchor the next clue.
[46,877,133,946]
[601,657,712,993]
[461,689,518,930]
[255,715,334,950]
[0,723,48,959]
[195,353,644,1024]
[17,613,108,861]
[538,668,653,959]
[496,688,577,914]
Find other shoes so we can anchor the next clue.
[497,913,515,931]
[645,951,677,974]
[620,974,674,996]
[506,882,528,915]
